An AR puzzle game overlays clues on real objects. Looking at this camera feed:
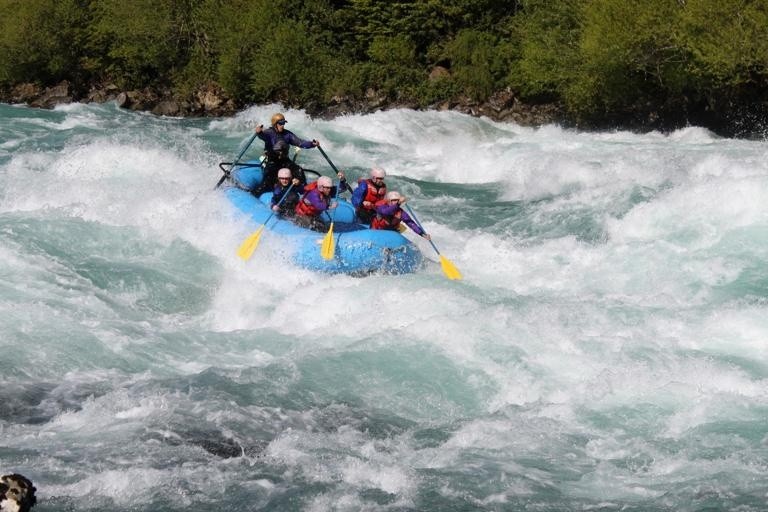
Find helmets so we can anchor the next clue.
[277,167,292,180]
[272,113,285,125]
[386,191,400,206]
[316,175,335,189]
[370,165,386,184]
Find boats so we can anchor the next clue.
[219,159,424,279]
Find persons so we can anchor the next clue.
[251,112,319,198]
[270,167,304,217]
[369,188,432,241]
[351,165,387,224]
[293,172,347,232]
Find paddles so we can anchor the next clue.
[403,202,463,281]
[320,176,340,261]
[236,182,296,260]
